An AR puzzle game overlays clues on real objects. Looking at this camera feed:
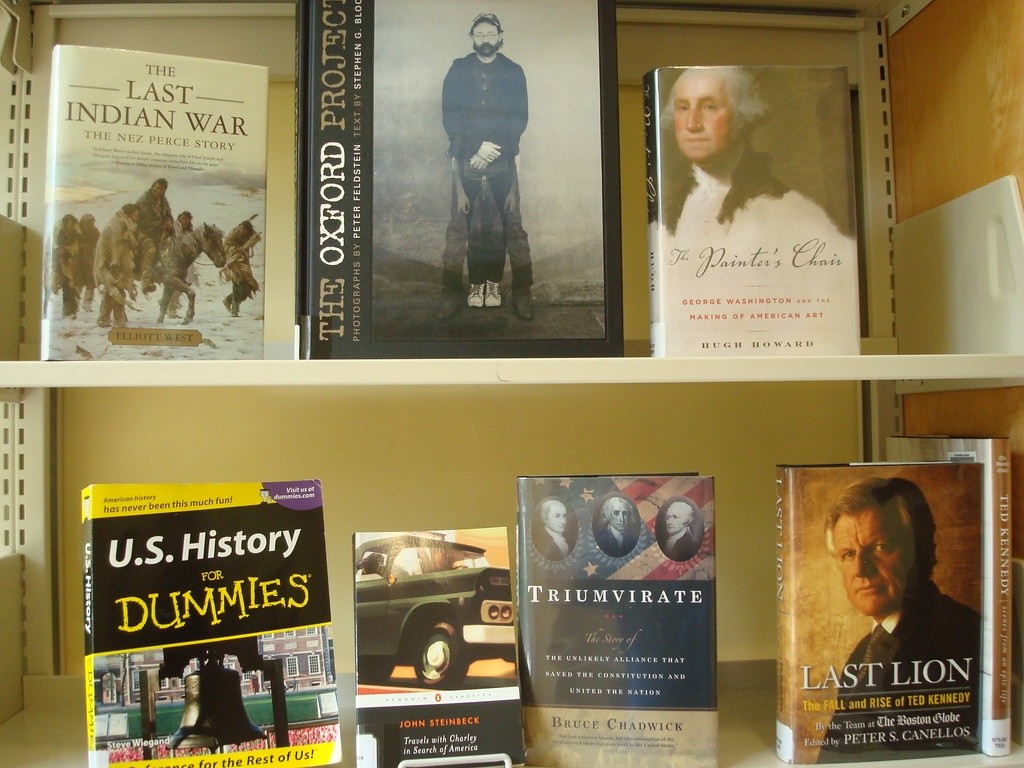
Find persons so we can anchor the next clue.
[535,497,696,561]
[436,12,533,322]
[660,66,782,236]
[816,477,982,765]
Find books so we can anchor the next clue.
[352,527,525,768]
[40,44,269,361]
[644,64,860,356]
[81,480,343,768]
[294,0,626,359]
[775,435,1014,765]
[517,472,717,768]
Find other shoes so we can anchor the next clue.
[437,295,466,323]
[515,291,534,320]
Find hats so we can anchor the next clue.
[469,12,502,32]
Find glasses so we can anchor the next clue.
[475,31,500,39]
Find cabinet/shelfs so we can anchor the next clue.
[0,1,1024,768]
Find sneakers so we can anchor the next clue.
[467,281,501,307]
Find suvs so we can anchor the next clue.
[354,530,517,691]
